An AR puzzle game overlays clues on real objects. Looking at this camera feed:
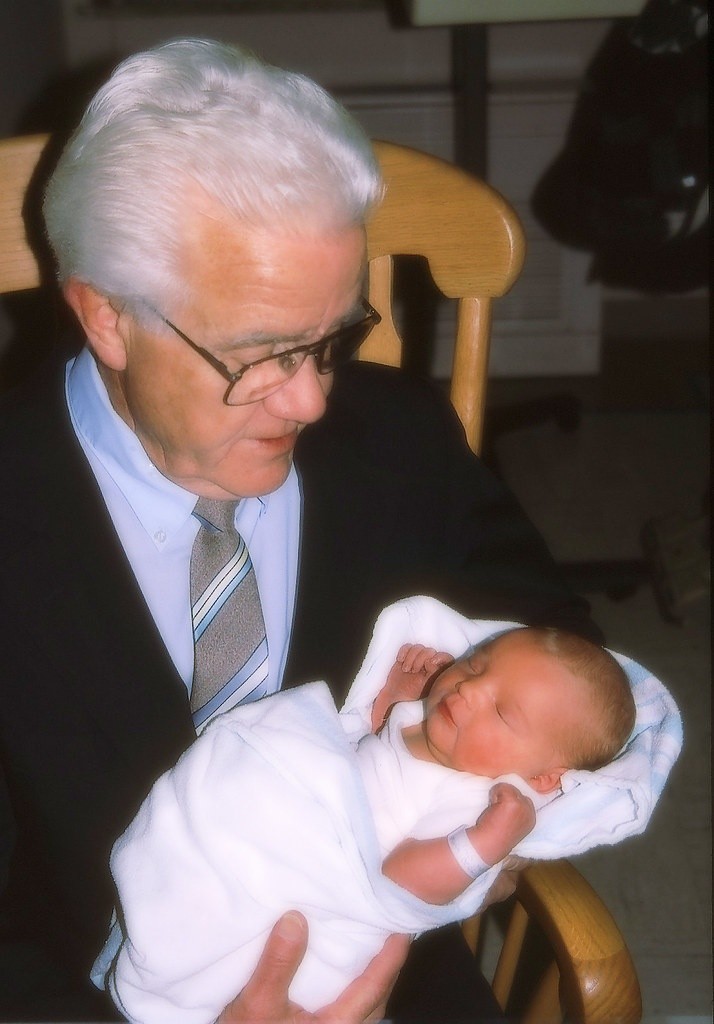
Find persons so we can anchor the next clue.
[136,626,637,939]
[0,41,605,1024]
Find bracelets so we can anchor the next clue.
[446,821,490,879]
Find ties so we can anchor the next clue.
[189,497,270,737]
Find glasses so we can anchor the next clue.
[147,296,382,406]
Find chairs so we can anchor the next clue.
[1,126,643,1024]
